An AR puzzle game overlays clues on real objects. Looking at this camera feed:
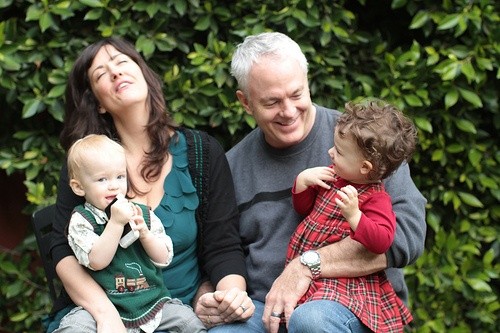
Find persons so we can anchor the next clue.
[278,97,419,333]
[34,40,256,333]
[192,32,427,333]
[50,134,207,333]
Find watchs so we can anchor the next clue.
[300,250,322,280]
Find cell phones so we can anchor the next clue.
[104,193,139,249]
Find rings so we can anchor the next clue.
[240,304,247,311]
[207,315,211,324]
[271,312,282,318]
